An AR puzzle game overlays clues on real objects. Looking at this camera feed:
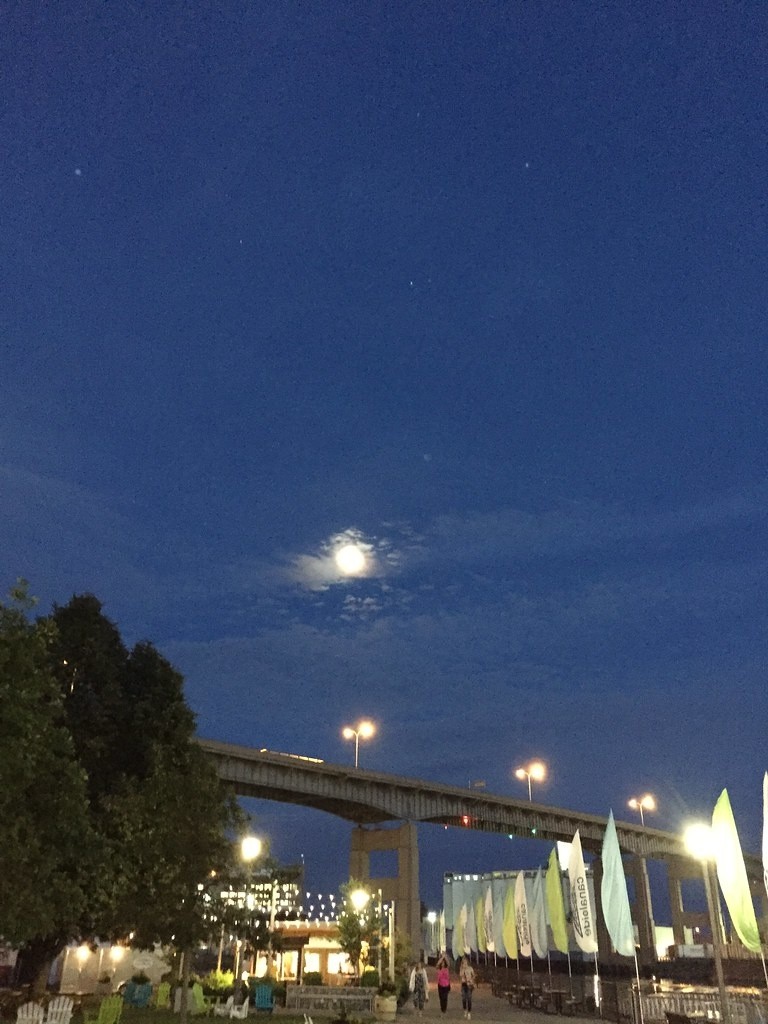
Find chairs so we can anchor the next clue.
[149,981,171,1010]
[188,982,248,1019]
[46,996,74,1024]
[84,995,124,1024]
[475,966,597,1017]
[256,984,276,1011]
[15,1001,44,1024]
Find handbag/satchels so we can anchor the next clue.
[425,992,429,1003]
[467,979,474,989]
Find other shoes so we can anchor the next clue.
[464,1010,471,1020]
[419,1011,423,1018]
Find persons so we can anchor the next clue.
[409,961,430,1017]
[436,950,453,1018]
[459,956,477,1020]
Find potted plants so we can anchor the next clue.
[373,982,398,1013]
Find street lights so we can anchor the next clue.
[630,795,654,827]
[344,721,374,766]
[516,763,545,800]
